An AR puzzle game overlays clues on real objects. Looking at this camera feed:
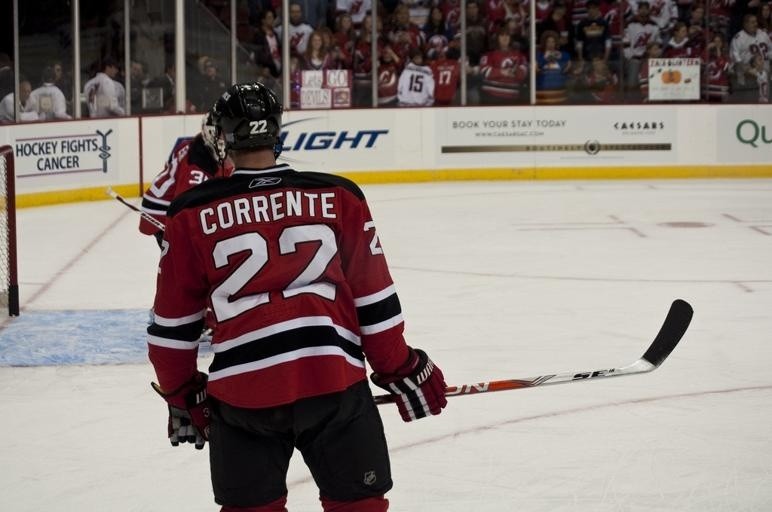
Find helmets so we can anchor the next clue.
[428,35,448,56]
[41,62,65,82]
[203,81,282,158]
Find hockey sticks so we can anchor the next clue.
[374,300,694,407]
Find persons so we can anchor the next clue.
[244,1,771,108]
[0,1,229,125]
[146,82,448,510]
[137,112,234,251]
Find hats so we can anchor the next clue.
[205,60,216,71]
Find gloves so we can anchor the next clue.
[151,371,213,449]
[371,349,448,422]
